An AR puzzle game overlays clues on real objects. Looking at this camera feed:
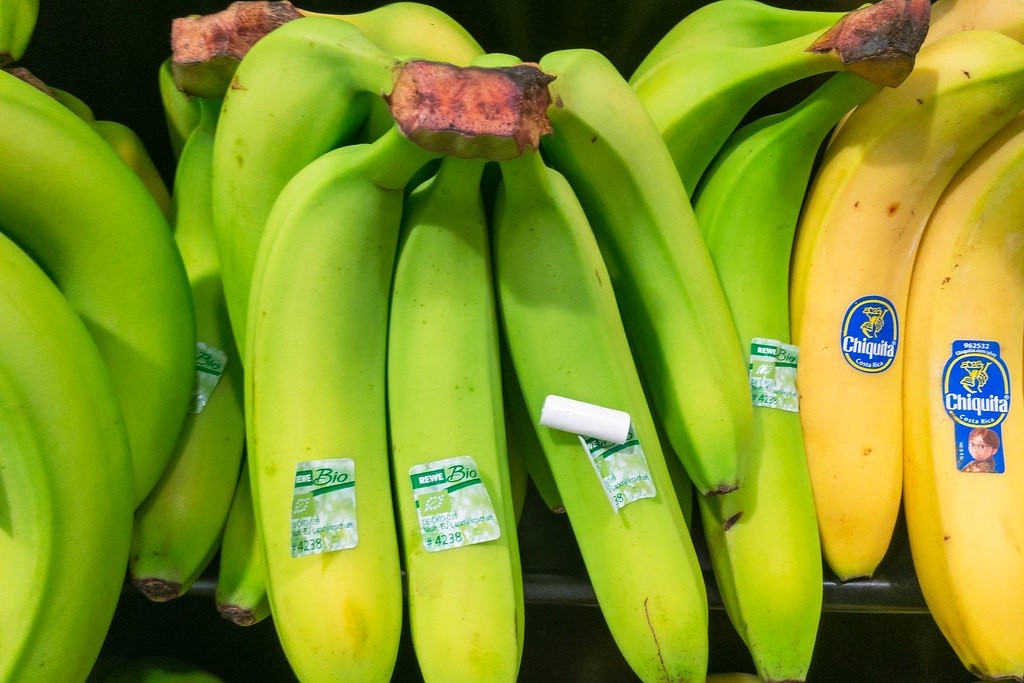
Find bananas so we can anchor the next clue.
[0,0,1024,683]
